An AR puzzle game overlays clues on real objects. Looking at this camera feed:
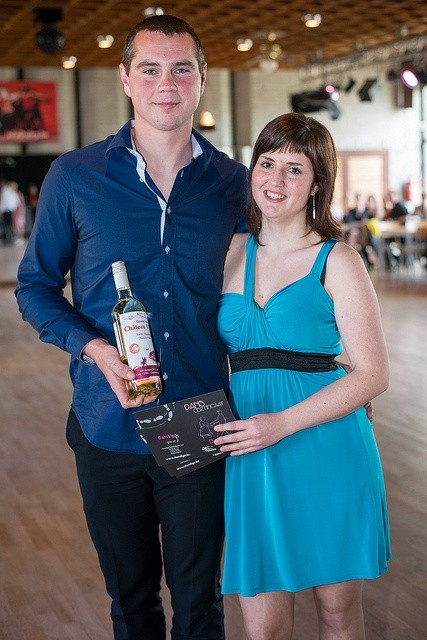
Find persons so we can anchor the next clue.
[214,112,393,640]
[14,15,374,640]
[0,181,427,268]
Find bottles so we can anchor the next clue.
[111,261,162,397]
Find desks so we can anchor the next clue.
[338,220,427,270]
